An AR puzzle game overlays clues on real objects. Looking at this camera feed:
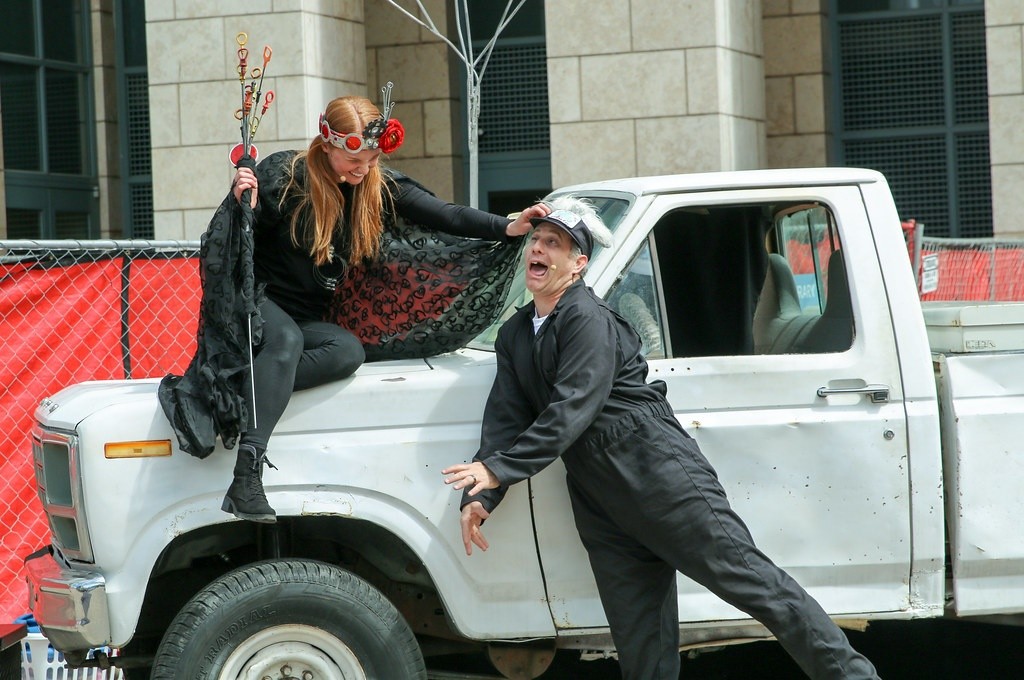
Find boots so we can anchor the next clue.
[221,444,278,523]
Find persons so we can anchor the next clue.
[158,96,553,523]
[441,196,880,680]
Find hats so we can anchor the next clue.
[529,208,594,261]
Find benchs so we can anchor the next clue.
[749,250,856,354]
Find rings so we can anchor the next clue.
[469,474,476,484]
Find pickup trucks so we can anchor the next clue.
[18,168,1024,680]
